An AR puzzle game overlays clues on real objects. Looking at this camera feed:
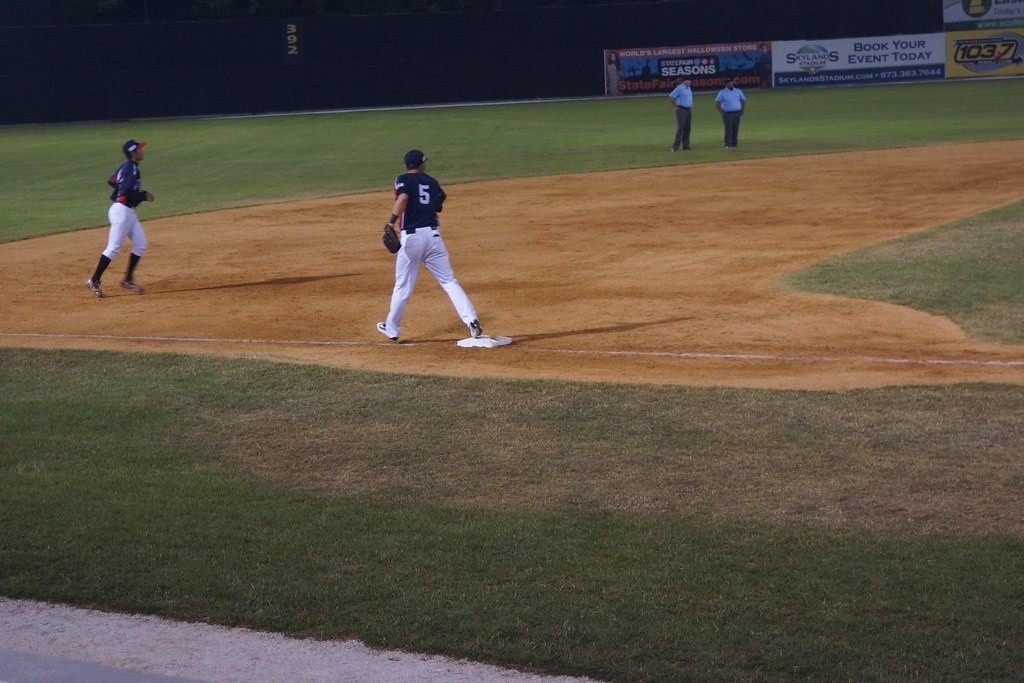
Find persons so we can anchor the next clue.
[376,149,484,340]
[715,77,746,148]
[668,75,694,153]
[641,59,653,92]
[754,44,773,86]
[85,137,156,299]
[605,51,626,95]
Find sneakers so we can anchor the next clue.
[470,319,482,339]
[86,279,103,297]
[377,322,399,341]
[120,279,144,292]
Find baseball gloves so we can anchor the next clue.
[380,223,402,255]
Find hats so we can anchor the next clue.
[123,140,145,156]
[405,150,428,165]
